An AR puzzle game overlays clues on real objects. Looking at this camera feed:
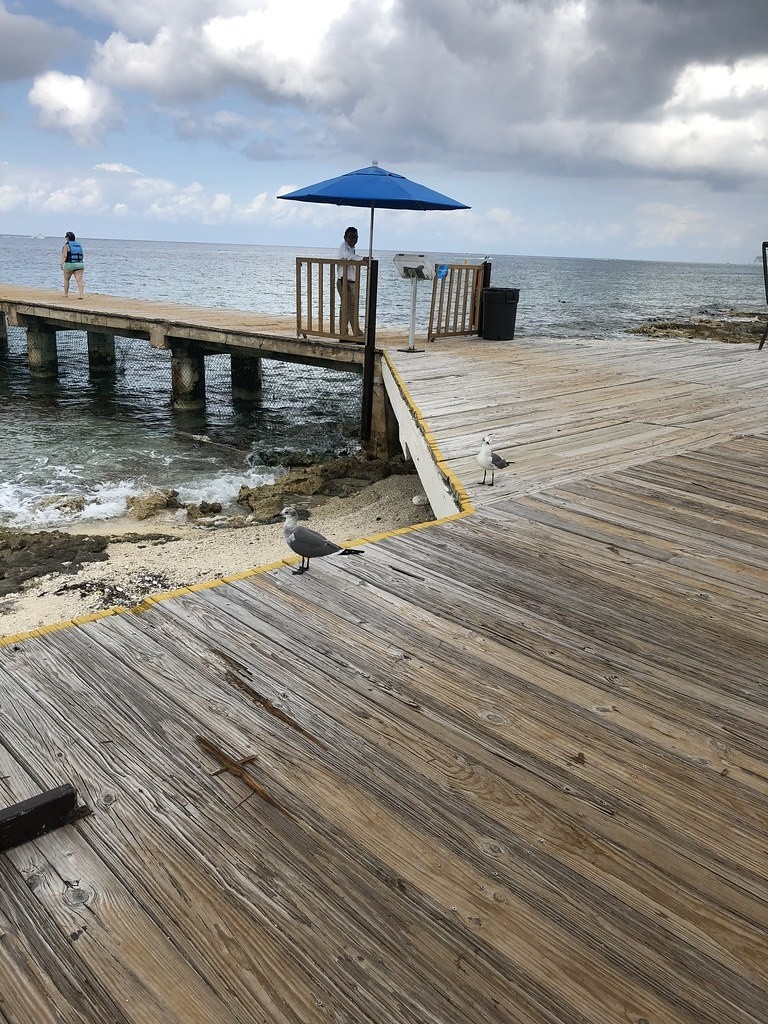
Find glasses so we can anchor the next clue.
[348,235,358,239]
[65,236,68,238]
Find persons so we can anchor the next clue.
[58,232,85,299]
[336,226,373,344]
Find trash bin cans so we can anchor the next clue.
[477,288,520,341]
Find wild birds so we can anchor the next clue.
[271,507,365,576]
[476,436,516,487]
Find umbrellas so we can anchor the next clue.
[278,159,472,345]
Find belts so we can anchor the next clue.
[339,279,355,283]
